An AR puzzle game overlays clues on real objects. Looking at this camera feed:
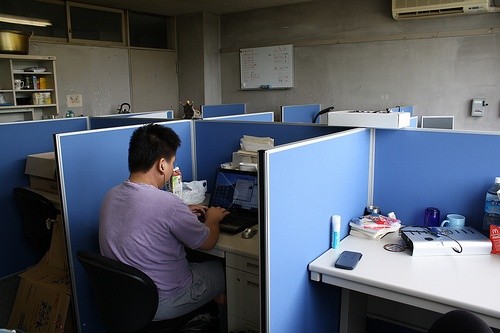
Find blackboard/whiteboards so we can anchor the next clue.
[239,44,294,91]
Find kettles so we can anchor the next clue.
[117,102,132,113]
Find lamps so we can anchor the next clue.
[0,13,52,27]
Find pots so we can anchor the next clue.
[0,28,34,56]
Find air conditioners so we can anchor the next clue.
[392,0,495,21]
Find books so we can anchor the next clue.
[349,212,401,240]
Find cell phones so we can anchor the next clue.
[335,251,362,269]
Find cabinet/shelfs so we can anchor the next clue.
[0,53,59,121]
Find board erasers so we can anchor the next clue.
[261,85,269,89]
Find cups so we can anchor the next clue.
[424,207,441,227]
[440,213,466,228]
[14,79,25,90]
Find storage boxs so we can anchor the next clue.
[25,152,59,194]
[30,76,46,89]
[167,176,182,198]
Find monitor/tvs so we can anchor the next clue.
[421,116,454,129]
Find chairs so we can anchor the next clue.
[14,188,61,234]
[76,250,221,333]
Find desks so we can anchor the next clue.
[14,185,60,210]
[196,224,260,333]
[308,232,500,333]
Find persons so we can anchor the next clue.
[99,122,230,321]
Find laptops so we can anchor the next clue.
[198,169,258,234]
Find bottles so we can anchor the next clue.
[482,176,500,231]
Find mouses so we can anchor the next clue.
[242,227,258,239]
